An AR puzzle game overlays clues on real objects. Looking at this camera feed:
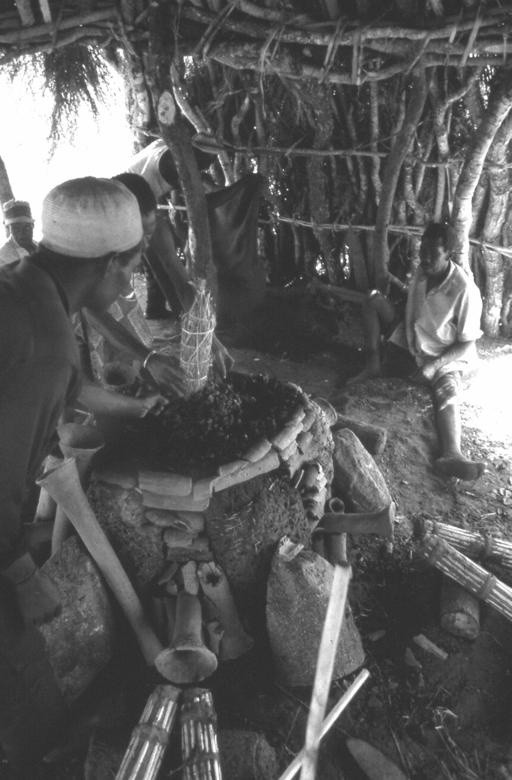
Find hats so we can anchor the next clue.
[1,198,37,229]
[38,175,145,263]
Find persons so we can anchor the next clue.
[345,223,485,481]
